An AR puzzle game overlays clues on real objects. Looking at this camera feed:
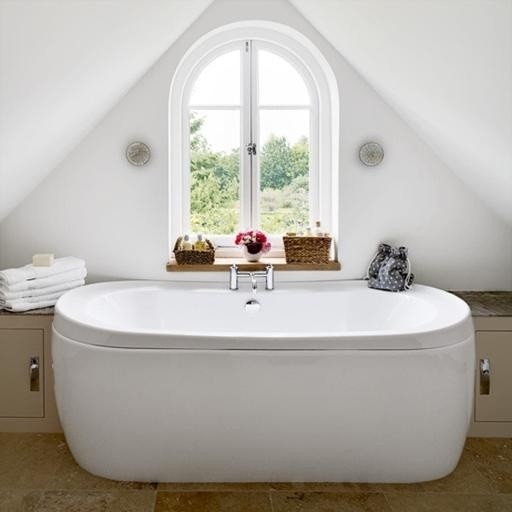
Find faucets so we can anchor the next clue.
[249,272,258,296]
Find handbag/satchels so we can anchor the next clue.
[366,242,414,290]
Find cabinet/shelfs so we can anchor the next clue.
[467,316,512,439]
[0,315,64,435]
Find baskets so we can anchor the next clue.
[172,235,215,265]
[282,235,332,265]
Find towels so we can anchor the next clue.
[0,255,87,354]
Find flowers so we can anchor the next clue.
[235,230,271,254]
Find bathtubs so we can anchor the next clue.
[52,281,475,483]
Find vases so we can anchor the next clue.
[243,242,263,262]
